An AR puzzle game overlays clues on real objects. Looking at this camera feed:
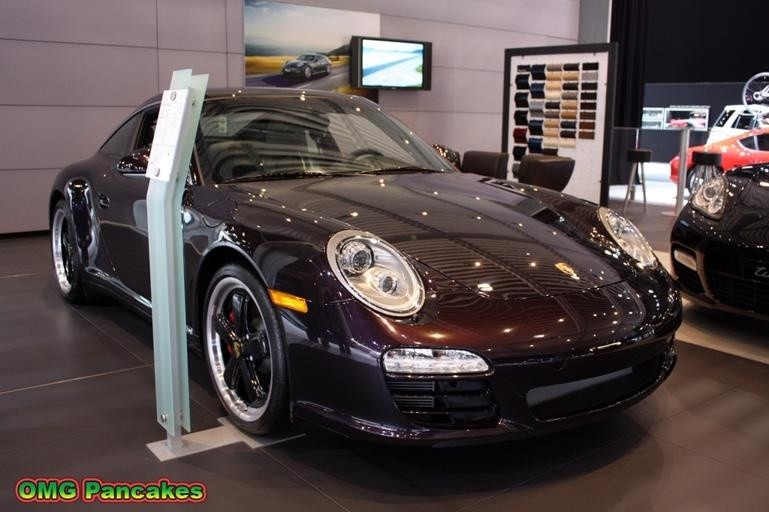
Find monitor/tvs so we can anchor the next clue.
[351,35,432,90]
[664,107,709,132]
[641,107,665,130]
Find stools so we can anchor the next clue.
[619,149,651,217]
[693,151,721,196]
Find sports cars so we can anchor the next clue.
[47,85,684,450]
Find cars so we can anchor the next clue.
[666,99,769,324]
[282,52,333,80]
